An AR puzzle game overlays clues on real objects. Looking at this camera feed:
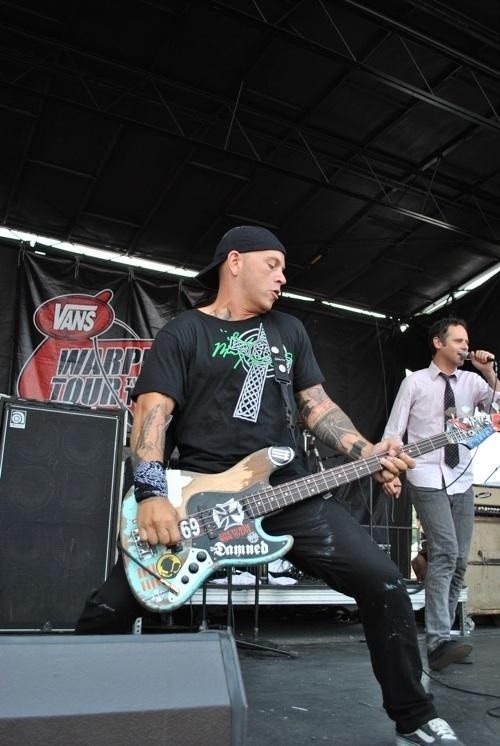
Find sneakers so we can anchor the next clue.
[427,639,474,670]
[396,718,465,746]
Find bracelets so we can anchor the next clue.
[134,461,168,502]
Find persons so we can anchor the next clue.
[380,318,500,670]
[75,225,468,746]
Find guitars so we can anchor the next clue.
[120,413,500,611]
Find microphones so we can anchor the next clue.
[462,352,493,362]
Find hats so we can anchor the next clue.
[195,225,287,289]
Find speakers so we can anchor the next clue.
[0,632,248,746]
[0,396,129,632]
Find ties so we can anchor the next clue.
[439,373,459,469]
